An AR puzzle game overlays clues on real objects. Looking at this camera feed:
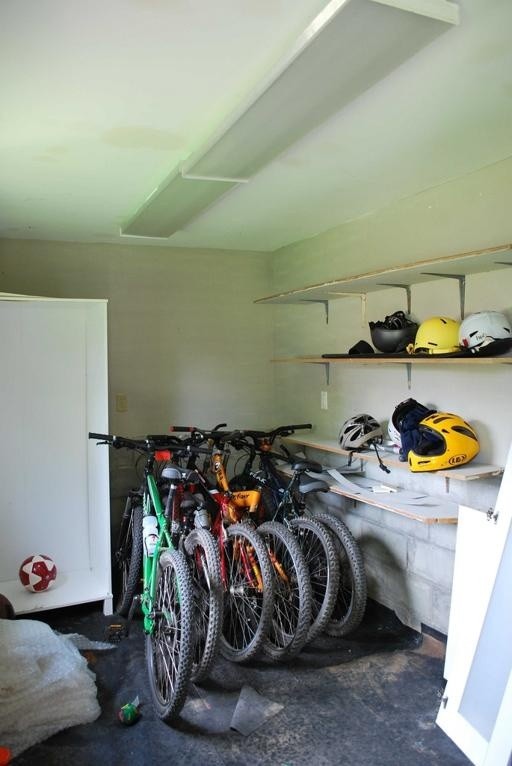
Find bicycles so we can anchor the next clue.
[145,431,244,687]
[168,422,369,663]
[88,432,223,724]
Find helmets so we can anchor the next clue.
[337,413,383,450]
[457,309,512,356]
[412,314,462,356]
[407,412,480,473]
[368,321,418,354]
[387,397,429,448]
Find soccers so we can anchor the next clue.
[119,704,139,725]
[20,555,57,593]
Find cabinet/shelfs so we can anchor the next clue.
[255,246,512,524]
[0,292,112,616]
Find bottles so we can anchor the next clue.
[142,515,159,558]
